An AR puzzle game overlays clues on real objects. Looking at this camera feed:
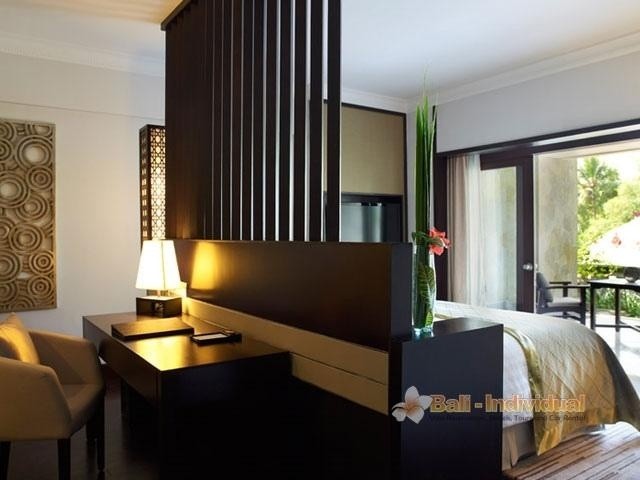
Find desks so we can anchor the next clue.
[70,306,306,480]
[590,269,640,328]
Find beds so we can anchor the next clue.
[157,238,611,479]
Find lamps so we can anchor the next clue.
[135,239,189,315]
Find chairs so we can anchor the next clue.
[532,270,585,321]
[1,313,105,480]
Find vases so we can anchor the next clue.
[414,248,436,335]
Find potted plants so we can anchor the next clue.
[410,84,451,252]
